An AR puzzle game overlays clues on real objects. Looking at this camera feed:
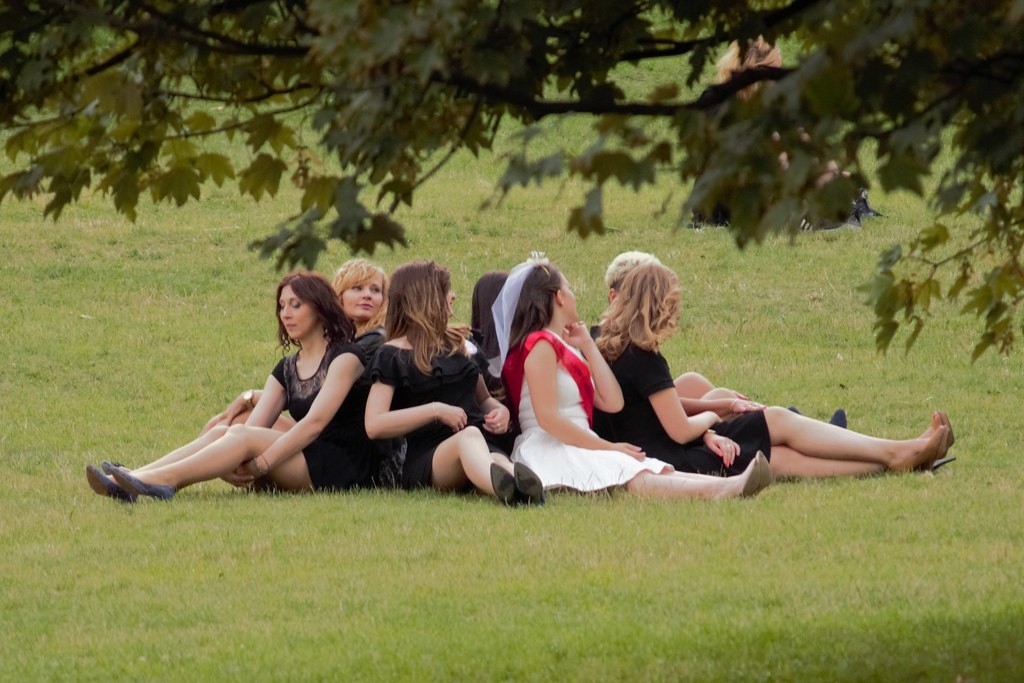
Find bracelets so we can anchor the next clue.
[253,454,270,476]
[729,399,738,410]
[432,402,437,425]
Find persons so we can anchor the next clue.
[691,35,868,231]
[86,251,957,508]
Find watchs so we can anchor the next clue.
[241,388,253,409]
[707,430,716,433]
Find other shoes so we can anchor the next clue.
[101,462,124,475]
[830,409,848,429]
[489,460,546,509]
[712,451,773,506]
[788,406,801,415]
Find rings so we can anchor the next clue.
[727,444,730,448]
[498,423,500,427]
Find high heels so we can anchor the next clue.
[885,410,956,475]
[85,462,176,507]
